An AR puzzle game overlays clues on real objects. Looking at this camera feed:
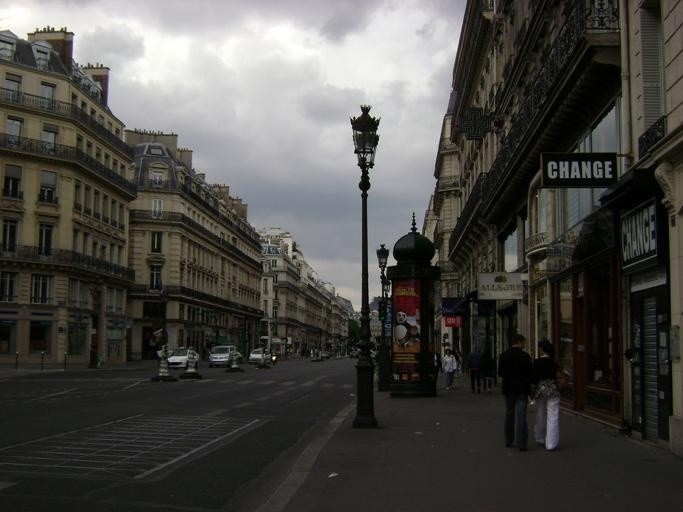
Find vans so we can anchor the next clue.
[209,345,236,367]
[249,349,271,364]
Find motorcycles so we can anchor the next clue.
[271,353,278,366]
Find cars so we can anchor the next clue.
[321,352,329,360]
[168,347,199,369]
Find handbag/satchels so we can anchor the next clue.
[535,380,556,398]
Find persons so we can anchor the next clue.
[476,346,496,394]
[440,348,456,390]
[467,349,480,394]
[496,333,532,451]
[529,339,561,450]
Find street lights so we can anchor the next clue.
[350,105,393,428]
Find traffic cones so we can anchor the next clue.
[225,346,244,372]
[255,349,270,369]
[179,345,201,379]
[151,345,177,382]
